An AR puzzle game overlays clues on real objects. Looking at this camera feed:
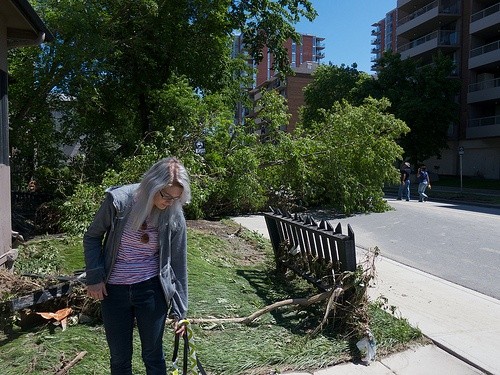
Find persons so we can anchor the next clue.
[417,166,430,203]
[83,158,192,375]
[397,162,410,201]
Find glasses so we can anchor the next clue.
[157,189,184,203]
[138,220,151,243]
